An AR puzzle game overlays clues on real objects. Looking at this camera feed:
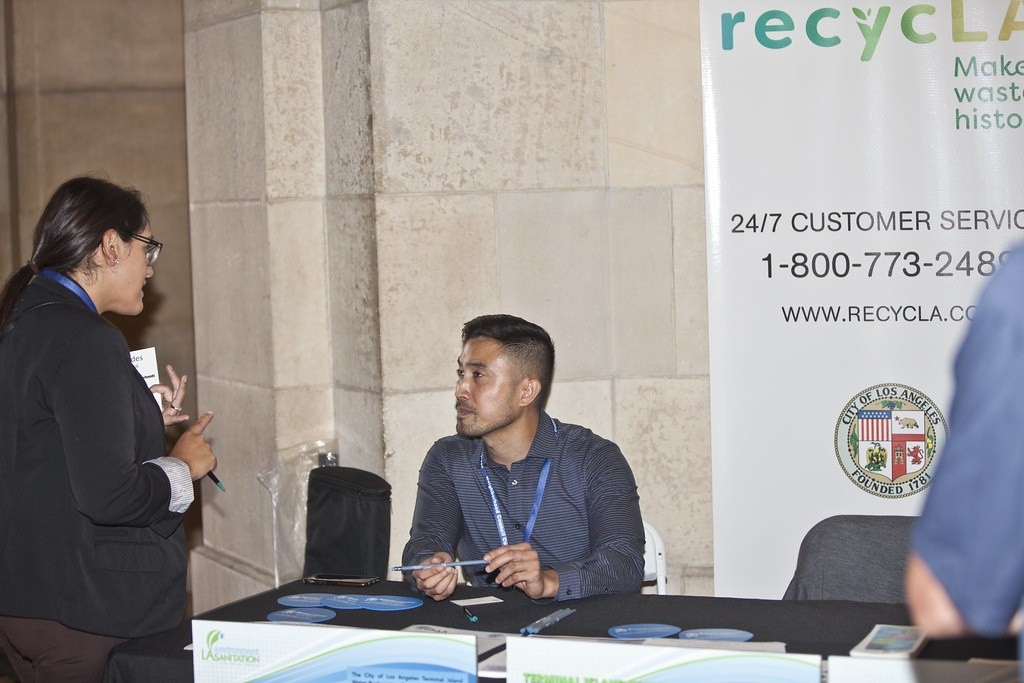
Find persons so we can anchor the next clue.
[1,175,218,682]
[400,313,646,605]
[903,236,1023,637]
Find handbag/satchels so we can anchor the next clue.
[302,466,391,580]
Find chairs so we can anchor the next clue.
[781,515,921,602]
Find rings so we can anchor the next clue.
[170,404,183,412]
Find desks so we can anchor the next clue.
[104,576,1021,683]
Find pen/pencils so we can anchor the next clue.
[462,605,478,623]
[519,607,578,634]
[207,470,227,492]
[392,559,492,571]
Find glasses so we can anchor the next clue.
[109,225,163,266]
